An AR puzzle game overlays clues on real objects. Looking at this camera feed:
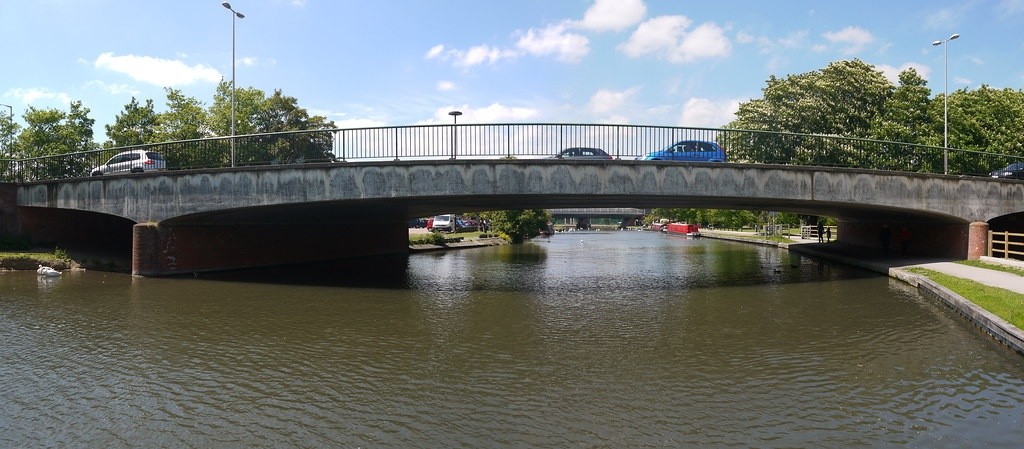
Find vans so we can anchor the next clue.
[407,217,428,228]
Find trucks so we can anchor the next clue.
[432,214,456,234]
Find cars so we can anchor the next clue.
[989,161,1024,180]
[549,148,612,159]
[634,139,729,163]
[427,218,434,232]
[89,148,167,177]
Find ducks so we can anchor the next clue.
[37,265,62,277]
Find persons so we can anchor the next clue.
[568,151,573,156]
[817,221,825,244]
[684,144,691,152]
[825,227,832,242]
[706,144,711,151]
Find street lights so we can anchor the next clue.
[223,2,245,167]
[449,110,463,158]
[931,34,956,176]
[0,103,13,180]
